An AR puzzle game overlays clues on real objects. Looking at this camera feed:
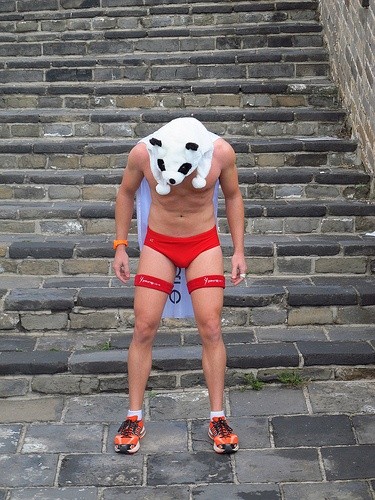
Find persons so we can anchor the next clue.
[113,117,246,455]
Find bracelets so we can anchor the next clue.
[114,239,128,249]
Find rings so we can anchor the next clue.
[240,274,246,277]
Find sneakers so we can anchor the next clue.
[115,416,146,455]
[208,416,237,454]
